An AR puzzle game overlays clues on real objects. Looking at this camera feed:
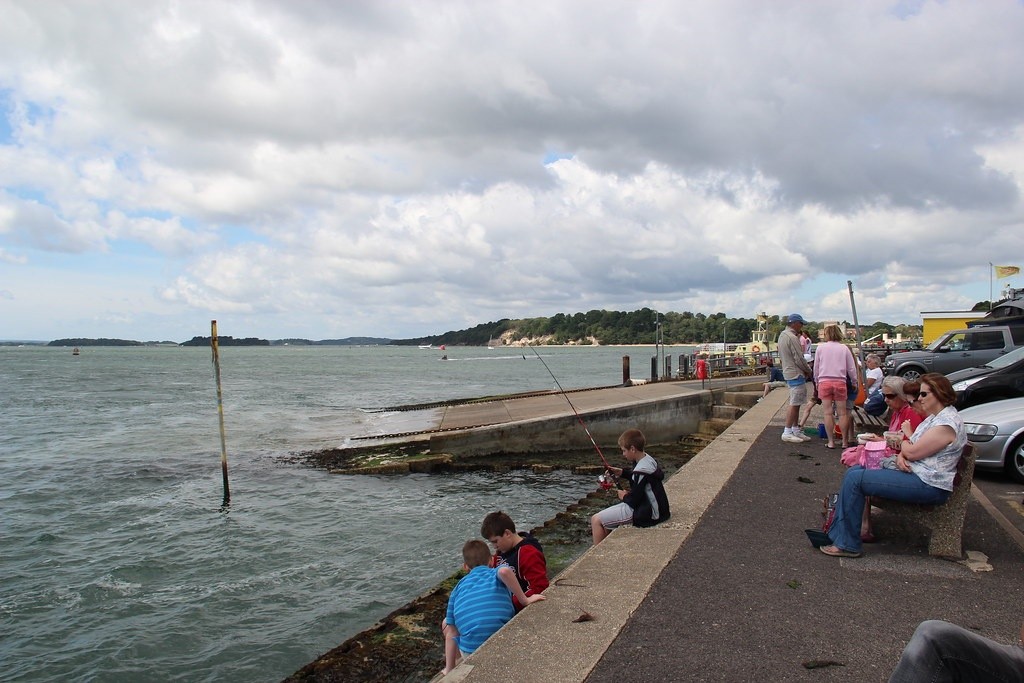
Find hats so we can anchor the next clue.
[788,313,807,325]
[804,353,814,363]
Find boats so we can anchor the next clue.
[695,311,779,367]
[72,346,80,356]
[440,345,445,350]
[441,355,447,359]
[418,344,432,349]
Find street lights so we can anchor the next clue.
[722,321,726,367]
[654,322,664,376]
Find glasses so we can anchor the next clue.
[918,391,932,397]
[882,393,898,400]
[908,398,919,408]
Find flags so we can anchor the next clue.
[995,265,1020,278]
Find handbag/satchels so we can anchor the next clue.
[822,493,876,543]
[864,430,904,471]
[864,388,888,417]
[854,378,866,406]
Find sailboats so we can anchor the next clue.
[488,334,494,349]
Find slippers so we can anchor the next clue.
[827,442,835,448]
[841,442,850,449]
[820,543,860,556]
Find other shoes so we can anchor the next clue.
[781,432,803,443]
[757,397,763,402]
[795,432,811,442]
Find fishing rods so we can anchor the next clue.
[519,337,624,491]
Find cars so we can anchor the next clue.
[943,345,1024,484]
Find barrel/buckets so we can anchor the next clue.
[817,423,828,438]
[834,423,842,438]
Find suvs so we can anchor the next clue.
[881,295,1024,382]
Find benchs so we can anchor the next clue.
[852,402,889,438]
[884,442,976,557]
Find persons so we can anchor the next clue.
[797,330,812,354]
[481,511,550,610]
[887,620,1024,683]
[441,540,546,675]
[798,390,822,430]
[821,373,968,556]
[591,428,671,546]
[757,362,786,403]
[778,313,813,442]
[813,325,860,448]
[865,353,883,395]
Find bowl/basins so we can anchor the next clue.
[883,431,905,450]
[857,434,874,444]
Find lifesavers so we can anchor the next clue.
[752,345,760,352]
[695,365,701,374]
